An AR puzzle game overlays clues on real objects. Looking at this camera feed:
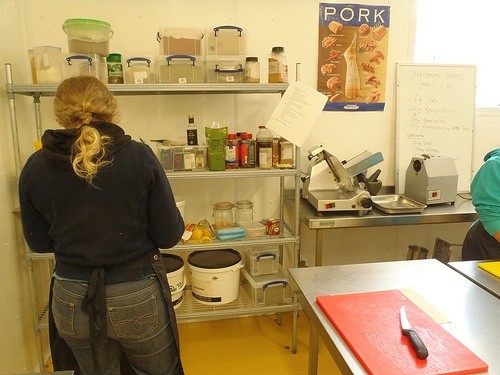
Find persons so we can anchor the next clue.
[470,148,500,243]
[19,76,187,375]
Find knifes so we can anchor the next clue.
[398,304,429,359]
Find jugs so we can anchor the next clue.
[33,45,62,85]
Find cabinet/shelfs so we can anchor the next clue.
[5,81,303,330]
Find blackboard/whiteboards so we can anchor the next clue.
[394,62,477,195]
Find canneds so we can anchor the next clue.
[244,56,260,83]
[267,47,288,83]
[223,132,256,169]
[265,218,281,236]
[107,53,124,84]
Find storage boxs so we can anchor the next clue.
[156,26,203,57]
[241,265,293,308]
[62,18,113,57]
[205,56,246,83]
[154,139,208,171]
[62,53,108,84]
[244,246,281,276]
[123,55,157,83]
[156,54,206,83]
[204,25,247,56]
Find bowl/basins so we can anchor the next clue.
[240,220,265,237]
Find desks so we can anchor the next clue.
[283,189,500,375]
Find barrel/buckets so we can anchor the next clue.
[188,248,245,304]
[161,252,186,309]
[61,18,113,54]
[174,195,186,227]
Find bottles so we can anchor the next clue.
[267,47,288,83]
[107,54,125,83]
[212,201,236,230]
[244,56,260,83]
[236,200,254,229]
[159,112,295,170]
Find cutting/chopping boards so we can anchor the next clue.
[316,289,489,375]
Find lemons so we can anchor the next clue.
[191,226,214,244]
